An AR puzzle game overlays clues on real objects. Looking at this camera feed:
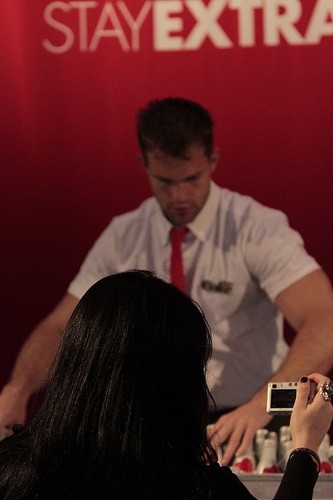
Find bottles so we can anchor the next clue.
[206,424,333,473]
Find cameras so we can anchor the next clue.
[266,381,315,415]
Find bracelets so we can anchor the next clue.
[289,447,322,473]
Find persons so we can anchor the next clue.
[1,95,332,466]
[1,269,333,500]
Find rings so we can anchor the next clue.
[316,379,333,401]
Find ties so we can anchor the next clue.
[169,224,189,293]
